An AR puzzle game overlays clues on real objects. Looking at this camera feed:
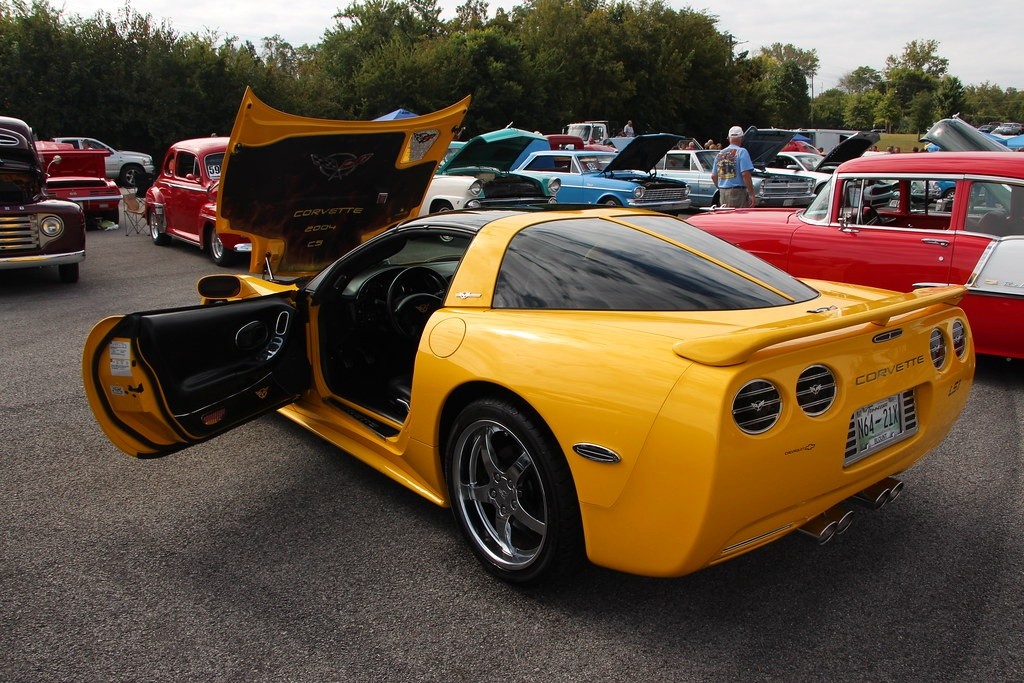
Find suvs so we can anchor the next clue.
[49,137,155,190]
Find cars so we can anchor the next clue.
[681,151,1024,361]
[34,140,124,226]
[0,116,86,284]
[145,133,253,268]
[414,111,1024,246]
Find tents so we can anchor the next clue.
[793,134,812,144]
[372,108,418,120]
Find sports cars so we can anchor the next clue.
[83,86,975,599]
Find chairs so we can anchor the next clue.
[123,193,152,237]
[967,211,1007,234]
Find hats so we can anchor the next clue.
[80,139,90,143]
[728,126,744,138]
[689,142,694,146]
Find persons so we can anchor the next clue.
[872,146,879,152]
[912,147,929,152]
[711,126,756,208]
[704,139,722,150]
[819,147,824,153]
[887,146,900,154]
[617,120,635,137]
[80,139,92,149]
[686,141,695,150]
